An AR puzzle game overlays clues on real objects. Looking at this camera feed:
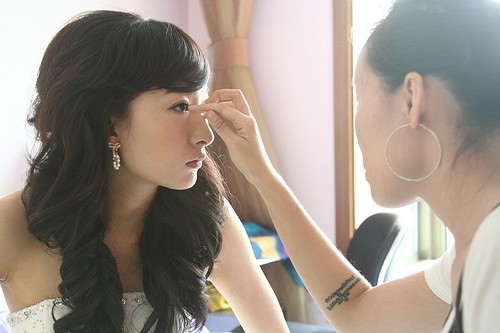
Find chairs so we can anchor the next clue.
[346,213,406,288]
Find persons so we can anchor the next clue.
[1,10,291,333]
[188,0,500,333]
